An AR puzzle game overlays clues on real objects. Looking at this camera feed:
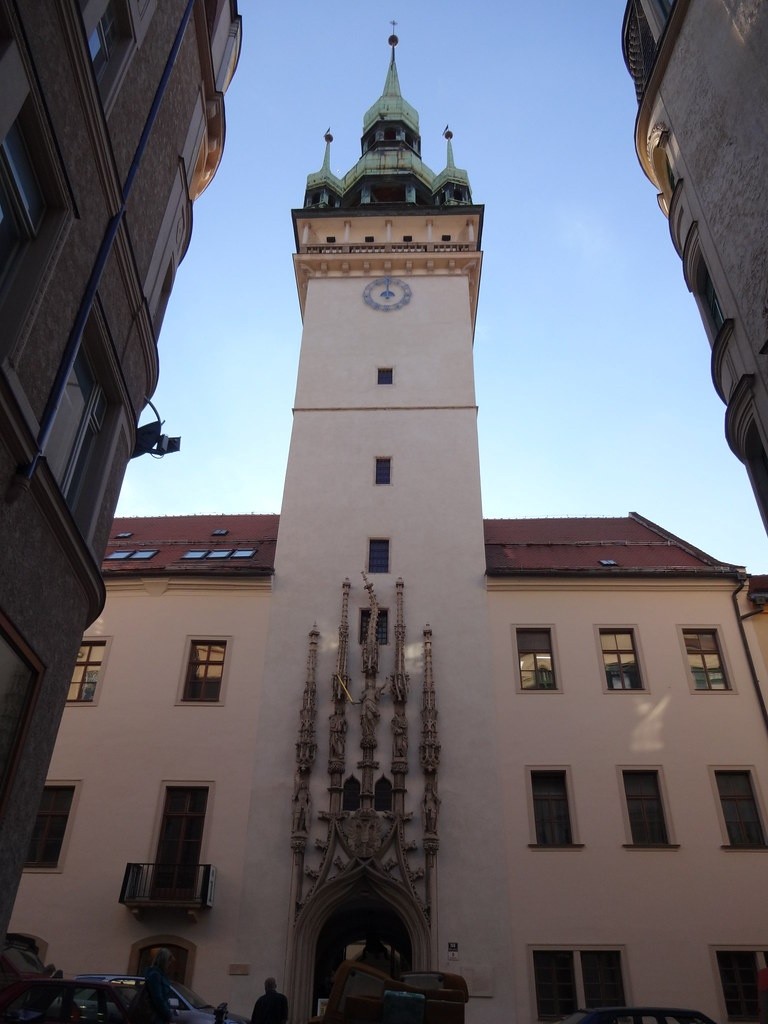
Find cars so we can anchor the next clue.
[0,933,252,1024]
[554,1006,719,1024]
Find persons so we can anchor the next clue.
[329,707,347,761]
[250,976,289,1024]
[290,779,311,835]
[421,783,441,834]
[350,675,387,735]
[391,709,408,756]
[144,947,175,1024]
